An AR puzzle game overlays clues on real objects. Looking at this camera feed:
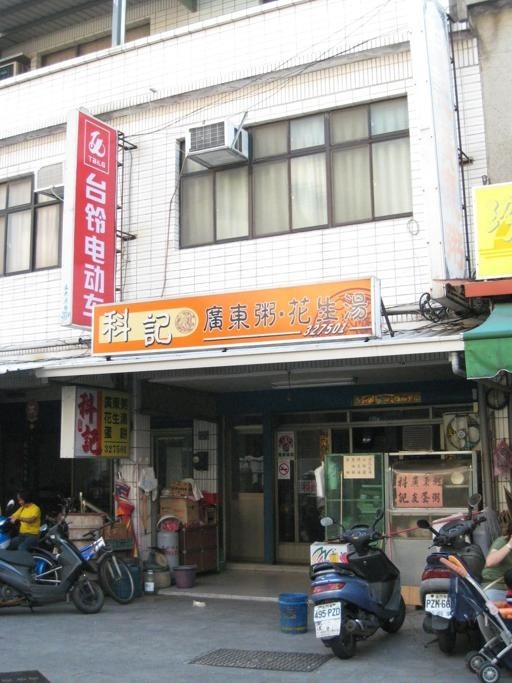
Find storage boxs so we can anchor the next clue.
[160,498,218,573]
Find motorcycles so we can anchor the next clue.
[0,498,106,613]
[414,493,488,655]
[309,509,407,659]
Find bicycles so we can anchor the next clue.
[29,517,135,604]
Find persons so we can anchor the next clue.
[8,489,41,552]
[485,522,512,603]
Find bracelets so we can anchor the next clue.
[505,544,511,550]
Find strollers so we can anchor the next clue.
[439,553,512,681]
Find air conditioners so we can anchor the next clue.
[184,120,249,167]
[34,161,65,200]
[441,409,482,453]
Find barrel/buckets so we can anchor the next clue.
[173,565,197,588]
[278,593,308,633]
